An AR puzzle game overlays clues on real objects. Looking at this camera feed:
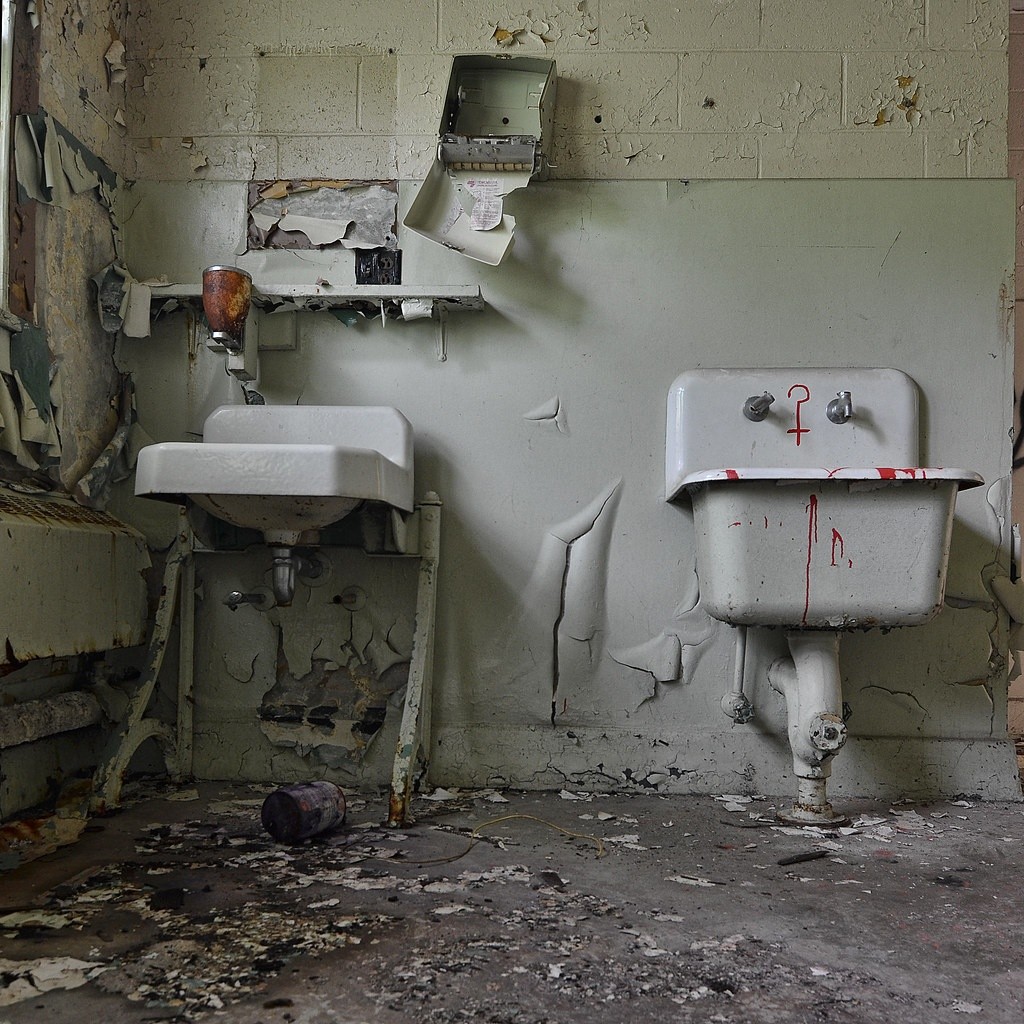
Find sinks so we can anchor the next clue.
[665,366,985,631]
[133,404,415,548]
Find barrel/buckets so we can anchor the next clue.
[261,780,347,844]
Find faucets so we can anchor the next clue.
[742,390,776,423]
[826,390,853,424]
